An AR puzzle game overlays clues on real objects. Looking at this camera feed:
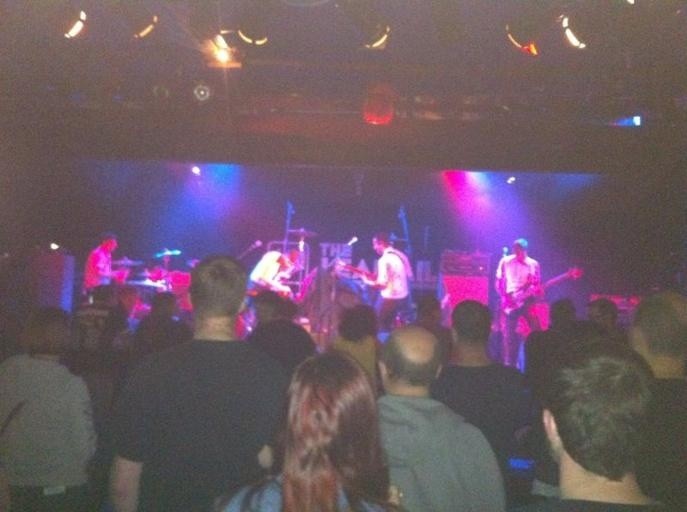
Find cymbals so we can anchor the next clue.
[287,229,323,238]
[112,257,146,266]
[153,250,181,261]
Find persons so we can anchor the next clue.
[0,230,687,511]
[224,350,404,511]
[522,336,679,511]
[109,254,288,511]
[431,302,525,511]
[627,289,686,511]
[373,324,506,512]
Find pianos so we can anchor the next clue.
[590,293,639,312]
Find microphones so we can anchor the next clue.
[348,236,358,246]
[251,239,263,249]
[502,246,508,258]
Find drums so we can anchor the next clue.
[134,284,158,305]
[87,285,122,302]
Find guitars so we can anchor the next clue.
[501,266,582,316]
[336,259,377,281]
